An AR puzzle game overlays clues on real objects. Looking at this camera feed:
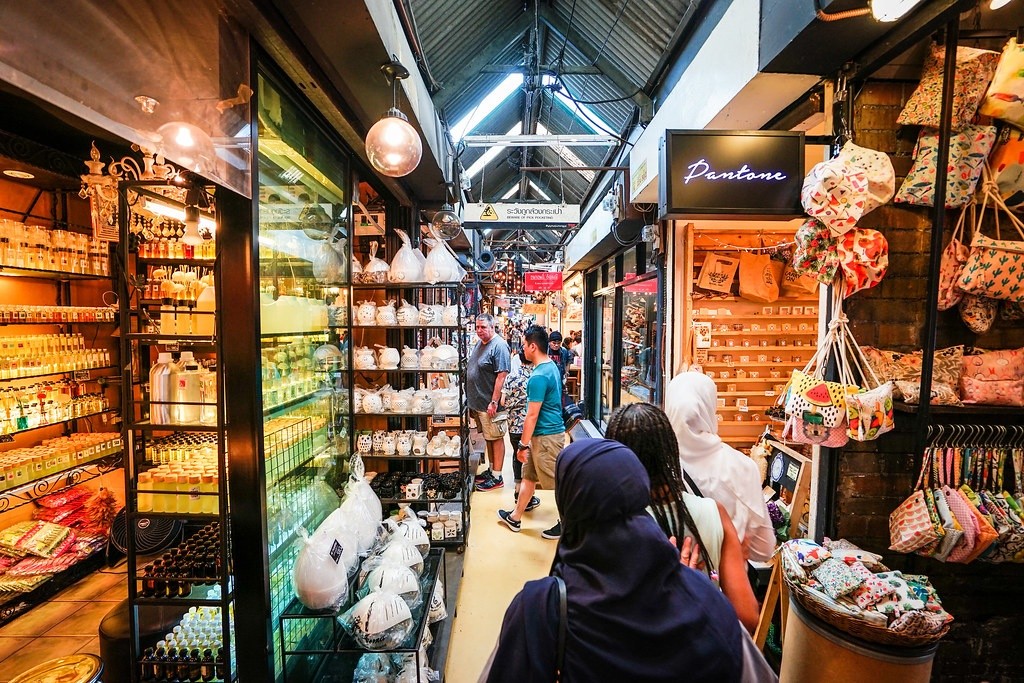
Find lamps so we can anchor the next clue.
[366,54,423,178]
[433,180,462,241]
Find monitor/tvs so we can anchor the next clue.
[665,128,806,214]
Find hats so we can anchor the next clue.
[790,143,895,298]
[549,332,562,342]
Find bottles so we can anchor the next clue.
[0,212,462,682]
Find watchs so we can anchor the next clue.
[491,401,498,405]
[518,440,530,451]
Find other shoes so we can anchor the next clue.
[514,493,540,510]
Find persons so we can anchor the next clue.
[496,317,582,512]
[497,324,566,541]
[475,439,777,683]
[639,333,658,390]
[605,403,763,632]
[464,314,514,491]
[660,371,776,563]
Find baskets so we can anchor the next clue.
[780,541,951,648]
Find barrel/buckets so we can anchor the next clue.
[776,591,935,683]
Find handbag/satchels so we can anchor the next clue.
[776,317,1024,565]
[562,394,583,432]
[935,165,1024,331]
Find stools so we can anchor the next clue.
[99,591,191,683]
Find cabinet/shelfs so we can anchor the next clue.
[1,153,471,683]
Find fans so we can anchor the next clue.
[106,499,184,568]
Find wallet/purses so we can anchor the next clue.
[785,537,954,636]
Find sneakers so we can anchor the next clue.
[475,468,492,482]
[542,519,562,539]
[475,473,504,490]
[498,510,521,531]
